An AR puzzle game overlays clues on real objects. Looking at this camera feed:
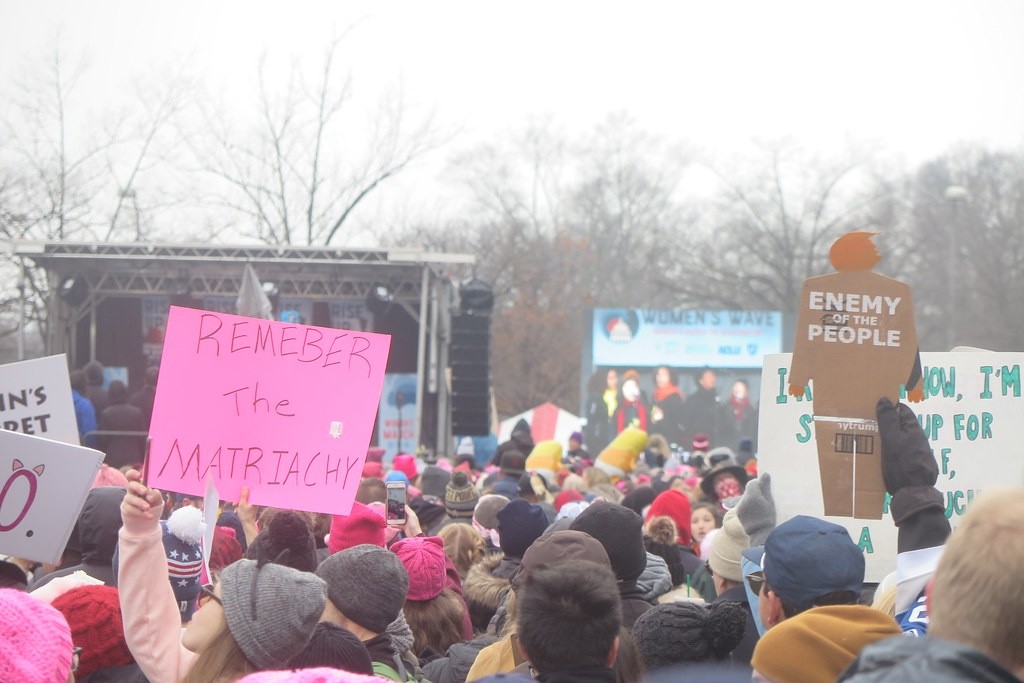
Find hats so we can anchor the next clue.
[0,419,1024,683]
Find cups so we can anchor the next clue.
[675,596,705,609]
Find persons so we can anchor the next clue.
[0,398,1024,683]
[70,359,160,445]
[587,365,759,453]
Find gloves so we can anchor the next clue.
[875,397,945,527]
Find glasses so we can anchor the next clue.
[704,558,713,575]
[249,556,275,620]
[70,646,83,672]
[744,570,769,596]
[195,583,224,610]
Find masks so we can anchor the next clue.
[715,477,740,500]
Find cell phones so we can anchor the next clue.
[385,481,408,524]
